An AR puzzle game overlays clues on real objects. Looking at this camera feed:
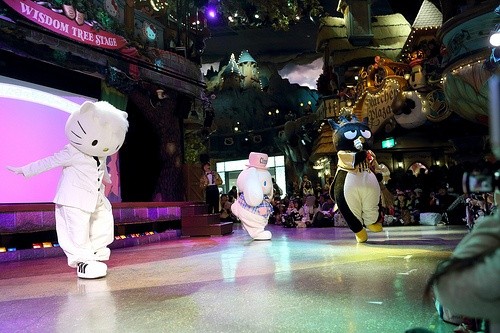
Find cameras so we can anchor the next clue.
[464,167,500,194]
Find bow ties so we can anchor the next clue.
[207,173,212,175]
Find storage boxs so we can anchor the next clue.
[334,213,348,227]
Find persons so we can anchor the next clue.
[380,161,497,230]
[218,181,338,229]
[300,174,312,188]
[199,156,223,214]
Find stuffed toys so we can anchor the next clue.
[327,114,390,243]
[230,151,274,241]
[5,100,128,279]
